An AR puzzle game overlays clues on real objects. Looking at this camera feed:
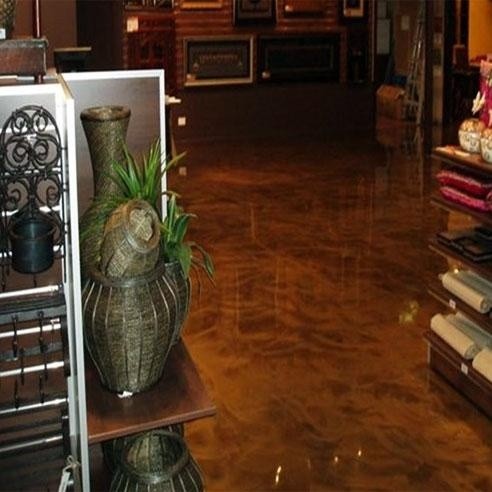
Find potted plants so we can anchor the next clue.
[80,124,218,348]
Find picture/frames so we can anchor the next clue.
[231,1,279,29]
[282,0,328,19]
[181,33,257,88]
[337,1,370,28]
[178,0,226,13]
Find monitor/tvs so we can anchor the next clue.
[53,46,96,72]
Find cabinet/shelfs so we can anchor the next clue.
[0,68,217,492]
[422,145,491,421]
